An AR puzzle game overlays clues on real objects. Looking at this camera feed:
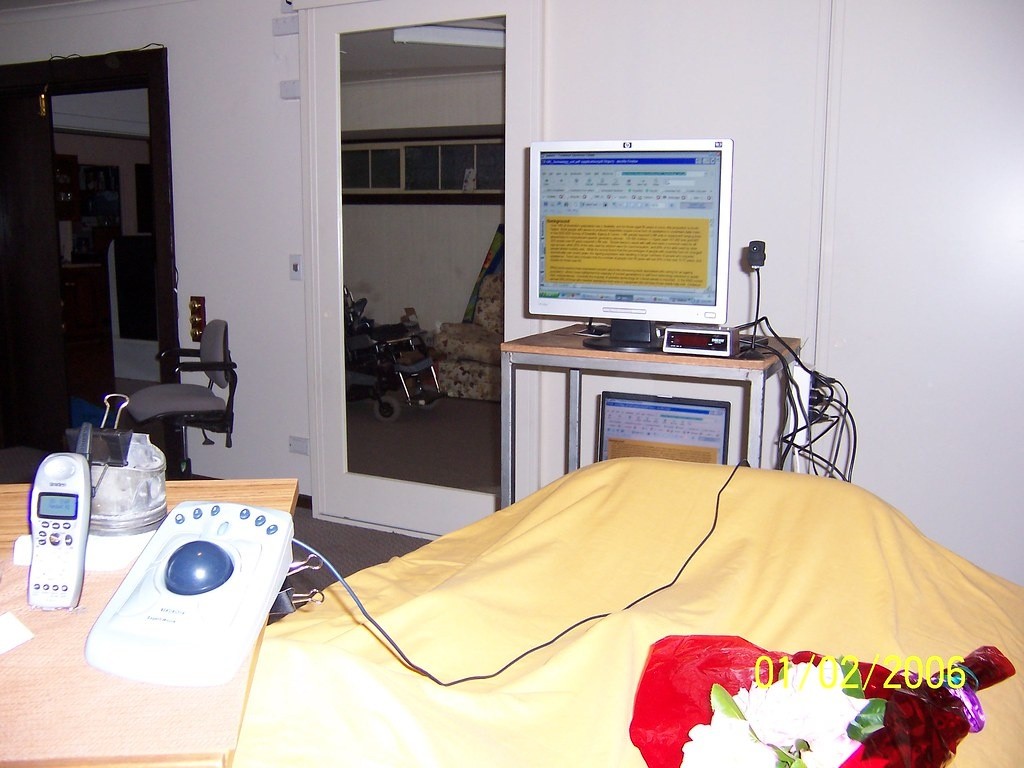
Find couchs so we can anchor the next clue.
[434,271,504,402]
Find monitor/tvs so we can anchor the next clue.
[528,138,734,354]
[597,390,731,464]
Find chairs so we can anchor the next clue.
[129,319,239,479]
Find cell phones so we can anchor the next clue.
[27,422,96,609]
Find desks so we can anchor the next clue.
[1,474,301,768]
[501,325,801,512]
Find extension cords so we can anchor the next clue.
[790,364,816,445]
